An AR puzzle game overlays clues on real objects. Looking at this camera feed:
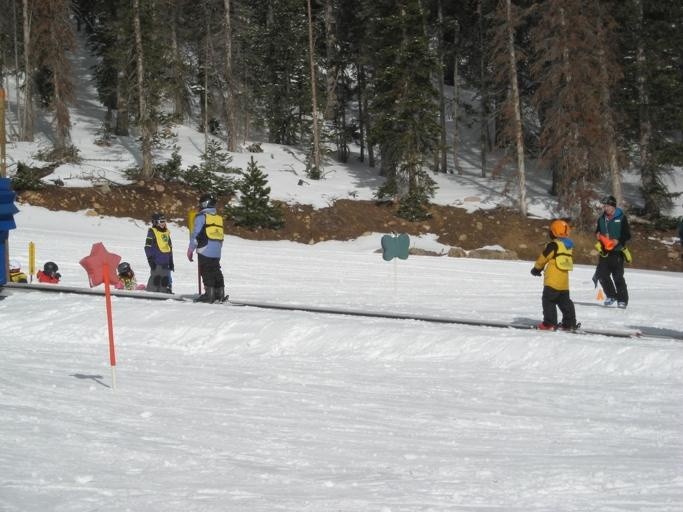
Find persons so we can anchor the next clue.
[10,260,28,283]
[115,263,144,290]
[37,262,61,283]
[187,193,224,304]
[531,219,576,332]
[144,212,174,293]
[595,196,630,309]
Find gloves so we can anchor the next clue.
[530,267,541,278]
[185,248,194,263]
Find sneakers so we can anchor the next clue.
[558,322,575,331]
[617,301,625,308]
[603,296,614,306]
[535,322,557,332]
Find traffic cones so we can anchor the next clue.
[595,288,604,302]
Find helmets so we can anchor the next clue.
[549,219,571,238]
[42,261,58,274]
[198,196,215,208]
[116,261,129,273]
[151,214,165,220]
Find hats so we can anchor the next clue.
[600,196,617,207]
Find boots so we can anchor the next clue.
[216,286,225,301]
[193,286,215,304]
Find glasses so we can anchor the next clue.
[157,221,164,224]
